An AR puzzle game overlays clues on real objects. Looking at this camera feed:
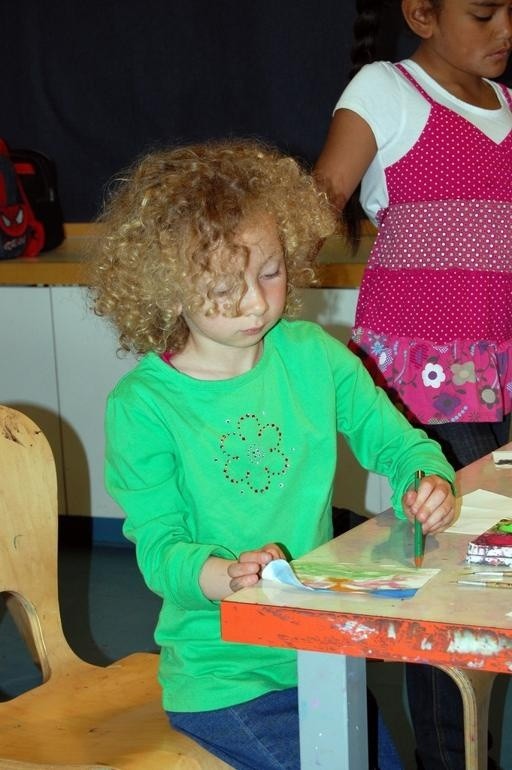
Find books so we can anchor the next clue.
[467,520,512,565]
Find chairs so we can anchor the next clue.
[1,402,225,770]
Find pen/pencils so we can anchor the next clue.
[414,470,425,569]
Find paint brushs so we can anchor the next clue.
[467,571,512,577]
[448,579,512,589]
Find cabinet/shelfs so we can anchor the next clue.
[47,288,360,548]
[1,286,66,554]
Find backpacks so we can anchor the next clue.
[0,138,66,260]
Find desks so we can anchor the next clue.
[220,437,512,769]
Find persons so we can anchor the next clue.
[306,0,511,463]
[85,141,456,769]
